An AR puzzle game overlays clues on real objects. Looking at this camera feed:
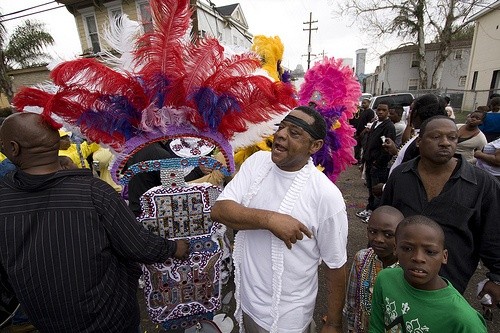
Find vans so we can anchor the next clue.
[367,93,415,124]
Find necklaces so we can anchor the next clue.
[359,252,377,315]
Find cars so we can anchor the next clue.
[358,93,373,106]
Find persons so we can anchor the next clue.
[355,102,396,223]
[0,130,273,219]
[355,99,375,168]
[381,116,500,321]
[343,206,404,333]
[373,94,500,195]
[304,100,345,185]
[368,215,488,333]
[0,112,193,333]
[210,105,349,333]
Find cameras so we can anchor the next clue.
[482,306,493,321]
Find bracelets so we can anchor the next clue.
[492,280,500,285]
